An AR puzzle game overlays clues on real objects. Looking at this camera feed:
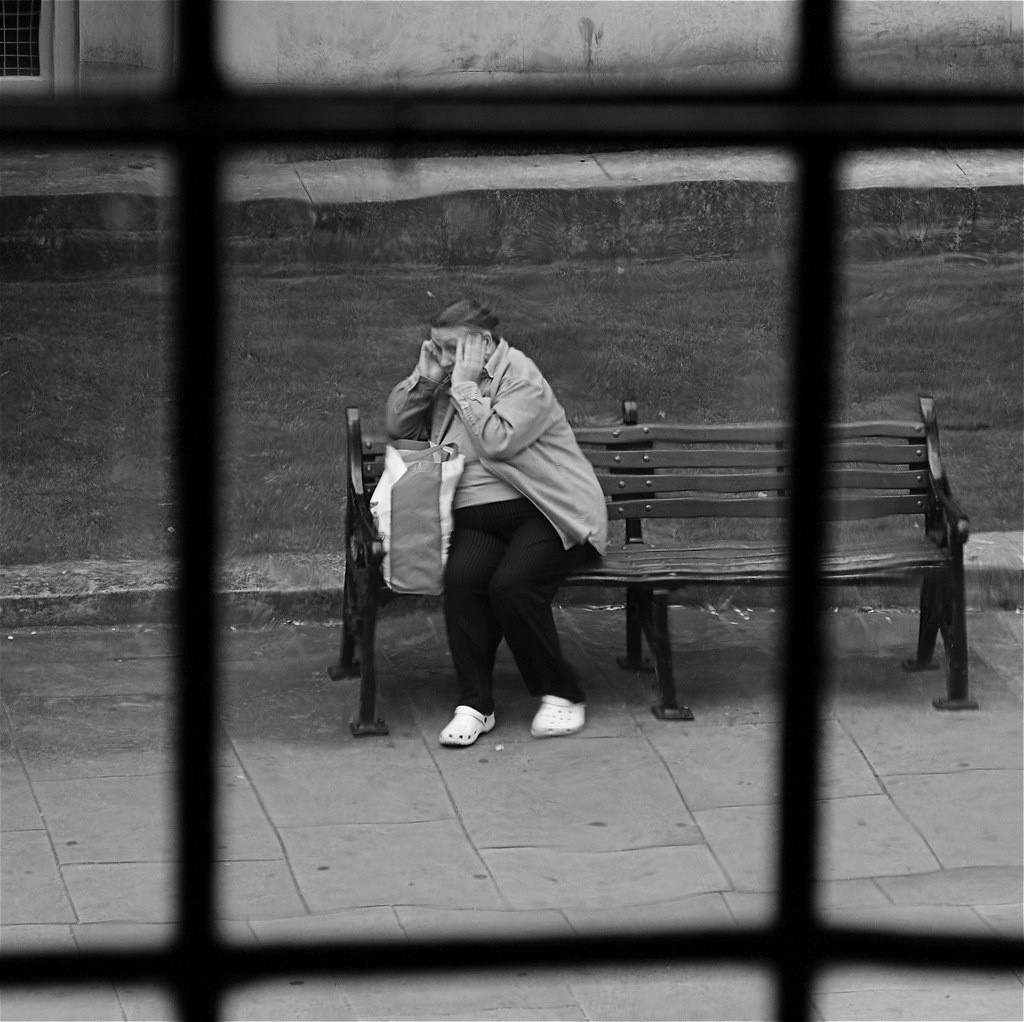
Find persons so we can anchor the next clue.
[386,299,608,747]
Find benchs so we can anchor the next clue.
[325,403,979,750]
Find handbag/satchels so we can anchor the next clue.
[370,440,465,596]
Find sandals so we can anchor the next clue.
[530,695,585,738]
[438,706,496,745]
